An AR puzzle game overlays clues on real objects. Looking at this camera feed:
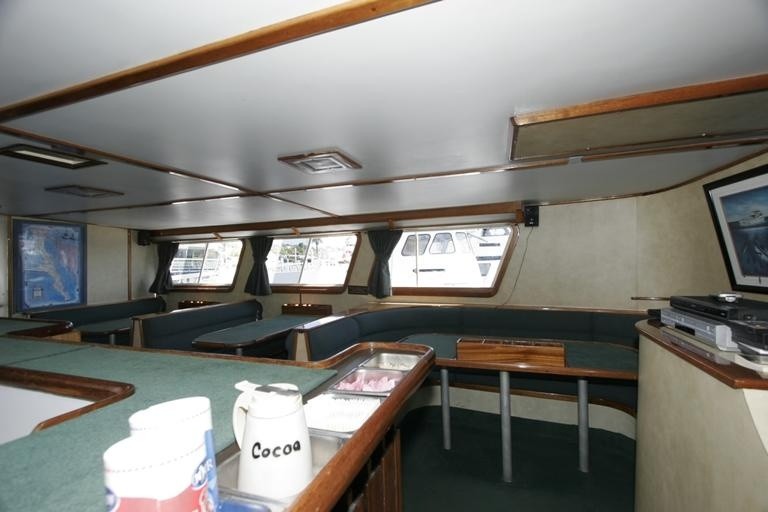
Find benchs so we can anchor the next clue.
[135,300,265,357]
[28,297,167,344]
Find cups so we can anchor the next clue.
[102,397,222,512]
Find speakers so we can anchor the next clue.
[524,206,540,227]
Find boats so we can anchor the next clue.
[739,209,767,229]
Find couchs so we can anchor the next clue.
[300,303,646,442]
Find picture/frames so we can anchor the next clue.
[702,163,768,294]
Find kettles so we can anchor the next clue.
[233,380,314,500]
